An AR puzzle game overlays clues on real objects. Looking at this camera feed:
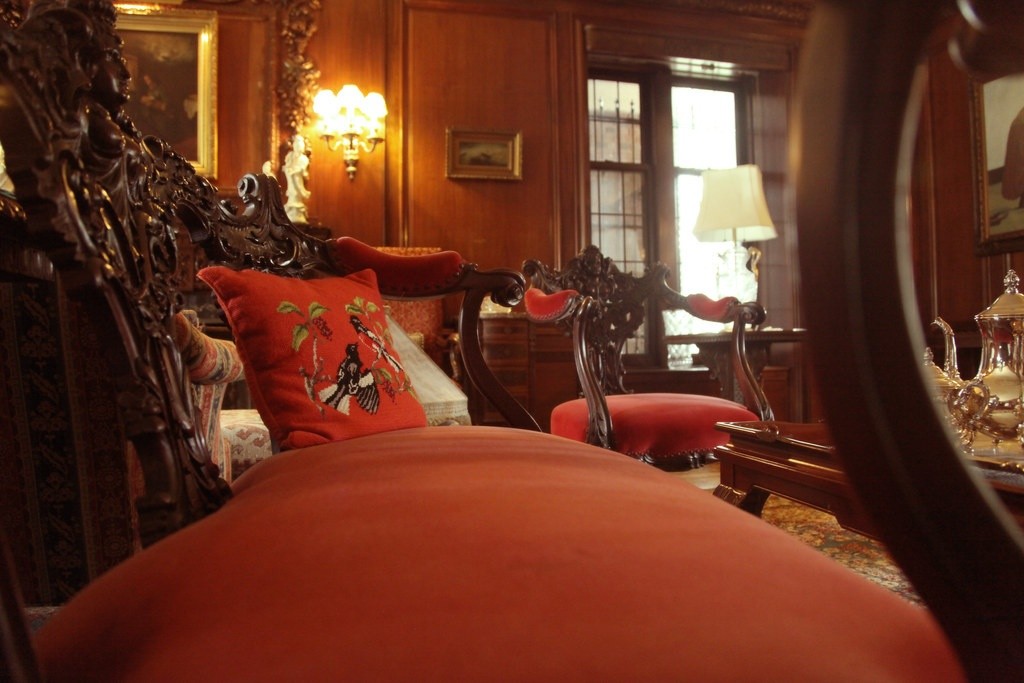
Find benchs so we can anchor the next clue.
[1,0,1024,683]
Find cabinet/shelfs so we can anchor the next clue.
[477,314,578,434]
[625,366,790,423]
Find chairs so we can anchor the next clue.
[373,246,461,388]
[523,244,775,456]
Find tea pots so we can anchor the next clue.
[923,347,990,456]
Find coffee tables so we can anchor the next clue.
[713,421,1023,543]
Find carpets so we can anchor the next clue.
[704,488,929,611]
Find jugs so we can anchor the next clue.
[930,268,1024,459]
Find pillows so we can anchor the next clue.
[197,267,427,453]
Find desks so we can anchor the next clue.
[663,330,807,405]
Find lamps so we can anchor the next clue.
[695,165,779,332]
[313,84,388,182]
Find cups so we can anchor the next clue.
[482,297,511,314]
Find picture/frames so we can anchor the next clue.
[115,5,218,181]
[445,126,523,181]
[969,56,1024,256]
